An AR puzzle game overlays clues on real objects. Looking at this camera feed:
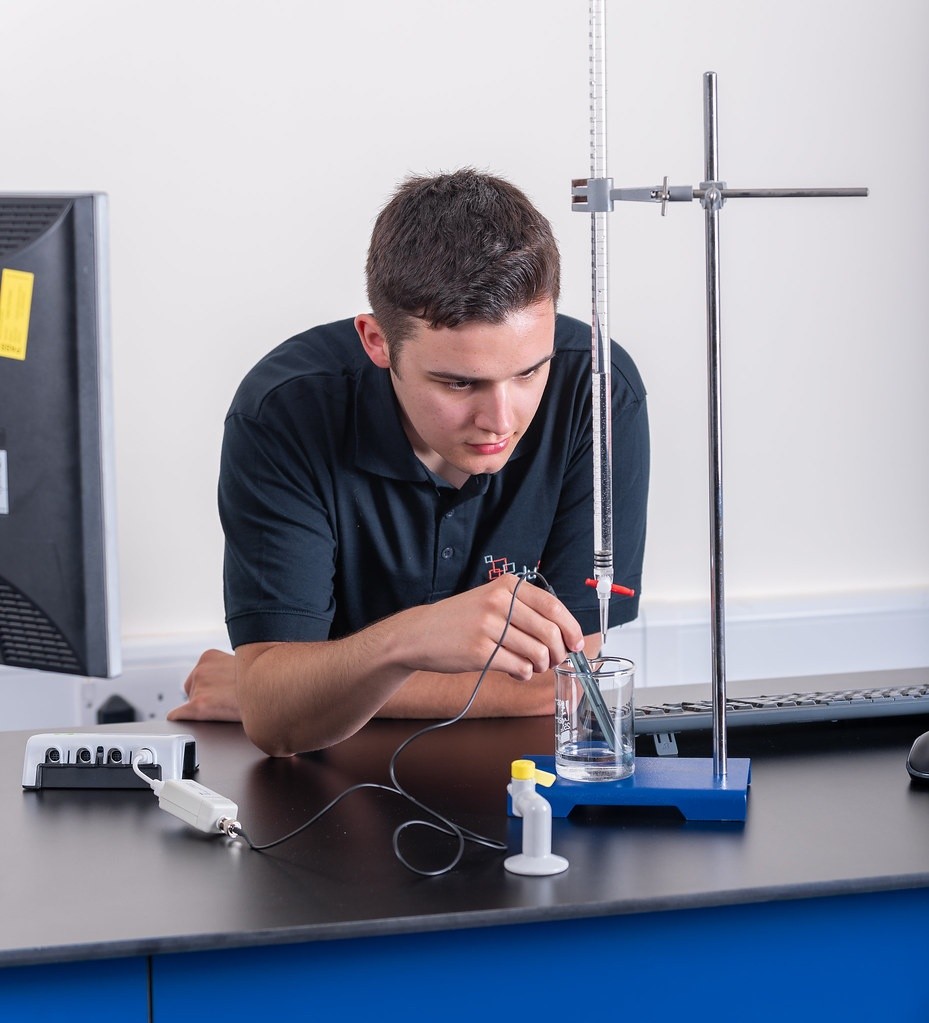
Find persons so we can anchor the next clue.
[167,166,650,757]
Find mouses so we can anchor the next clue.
[905,730,929,785]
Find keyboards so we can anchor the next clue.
[578,681,929,741]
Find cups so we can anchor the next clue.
[554,658,634,782]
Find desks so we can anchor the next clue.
[0,663,929,1023]
